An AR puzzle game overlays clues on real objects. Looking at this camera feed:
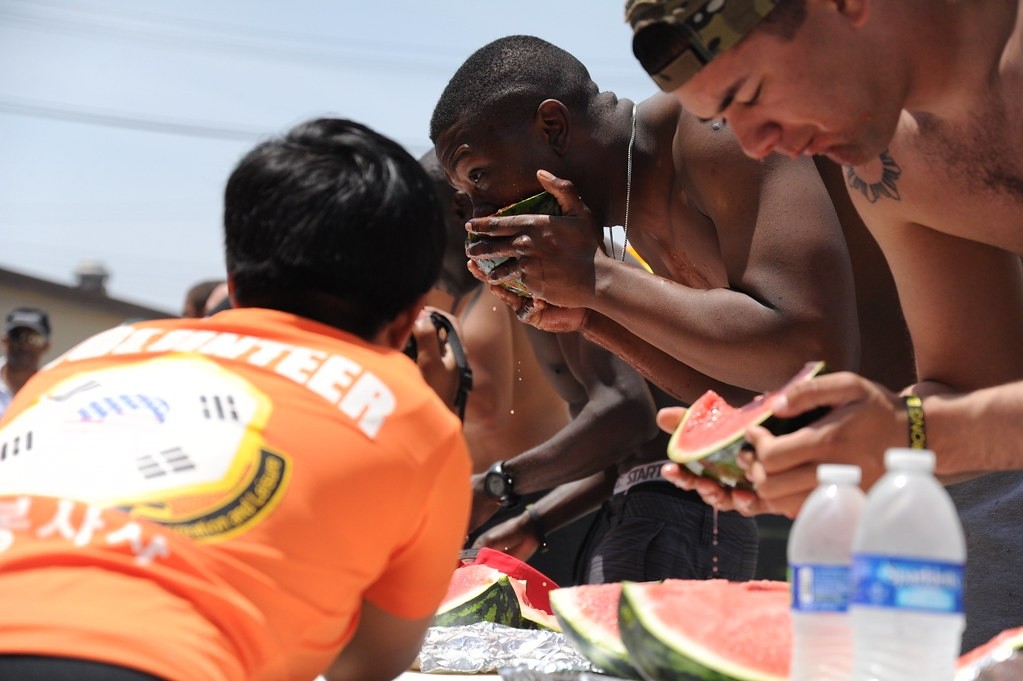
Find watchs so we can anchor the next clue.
[486,458,519,509]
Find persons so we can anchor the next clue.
[0,308,52,417]
[184,0,1023,657]
[1,119,476,681]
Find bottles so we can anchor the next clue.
[786,463,865,681]
[849,447,968,681]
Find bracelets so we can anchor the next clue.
[901,393,931,449]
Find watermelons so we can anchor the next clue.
[957,625,1023,670]
[667,361,828,490]
[432,565,561,631]
[548,579,793,681]
[467,192,560,301]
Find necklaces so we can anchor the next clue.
[610,103,638,265]
